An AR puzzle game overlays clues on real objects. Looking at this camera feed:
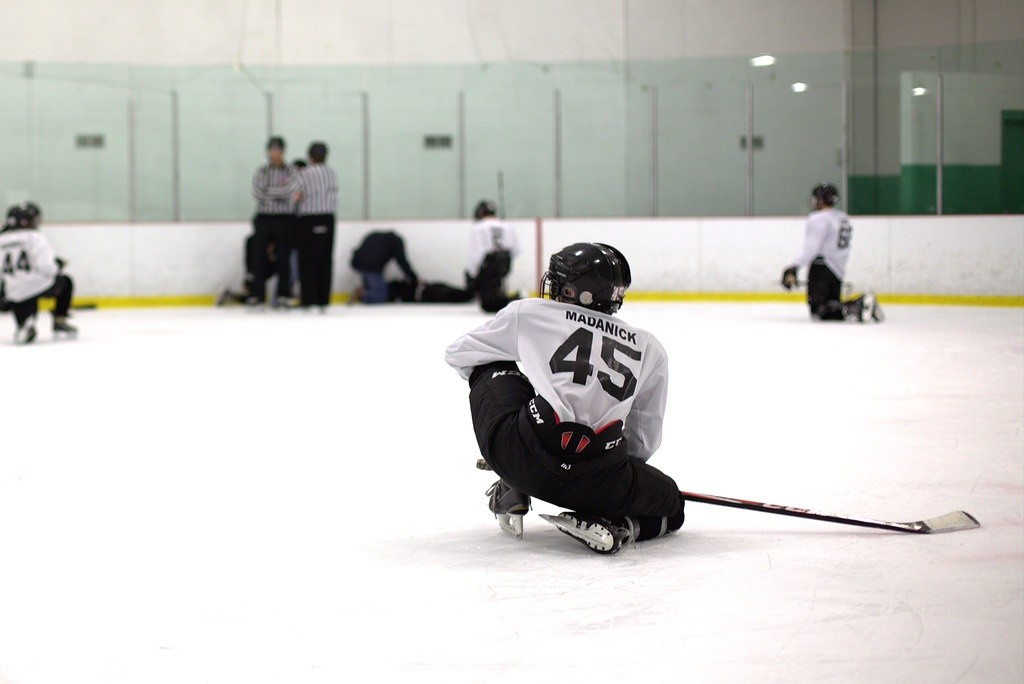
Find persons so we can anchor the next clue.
[779,180,888,325]
[217,136,529,314]
[0,203,78,345]
[446,241,685,554]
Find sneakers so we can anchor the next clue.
[847,290,874,322]
[15,319,34,342]
[486,478,531,539]
[539,511,635,555]
[52,314,77,340]
[873,295,886,322]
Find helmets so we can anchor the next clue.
[813,184,838,205]
[475,202,495,220]
[15,201,39,227]
[548,242,631,315]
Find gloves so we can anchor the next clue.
[782,267,800,289]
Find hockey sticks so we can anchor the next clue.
[680,491,980,535]
